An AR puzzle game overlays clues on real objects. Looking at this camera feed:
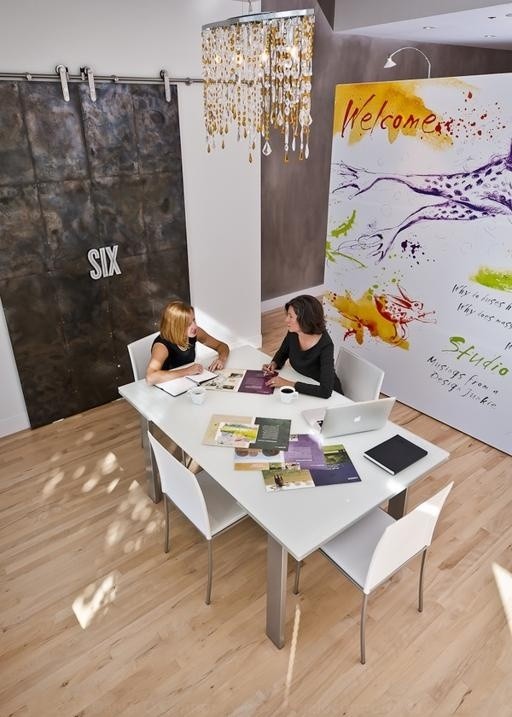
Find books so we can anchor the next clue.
[153,363,219,397]
[203,414,362,492]
[363,434,427,475]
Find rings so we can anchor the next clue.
[272,381,275,384]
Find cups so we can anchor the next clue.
[279,385,299,405]
[184,387,208,405]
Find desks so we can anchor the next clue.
[118,344,451,650]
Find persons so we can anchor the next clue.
[261,293,344,398]
[143,297,230,385]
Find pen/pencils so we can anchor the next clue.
[263,365,272,378]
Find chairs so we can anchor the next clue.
[147,431,248,605]
[127,331,160,449]
[293,482,453,663]
[335,344,385,402]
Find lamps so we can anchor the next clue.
[196,1,319,162]
[383,46,431,79]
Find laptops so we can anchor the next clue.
[301,396,396,439]
